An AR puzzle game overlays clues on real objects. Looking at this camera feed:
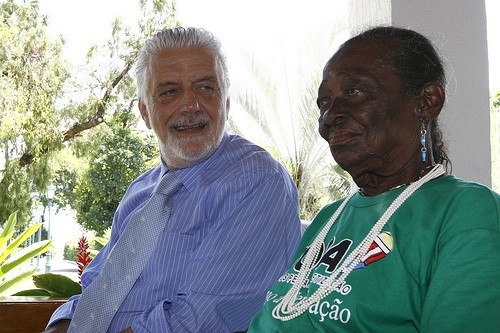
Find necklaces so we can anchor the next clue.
[271,164,445,322]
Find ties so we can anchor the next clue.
[66,168,185,333]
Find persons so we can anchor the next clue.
[246,26,500,333]
[39,27,301,333]
